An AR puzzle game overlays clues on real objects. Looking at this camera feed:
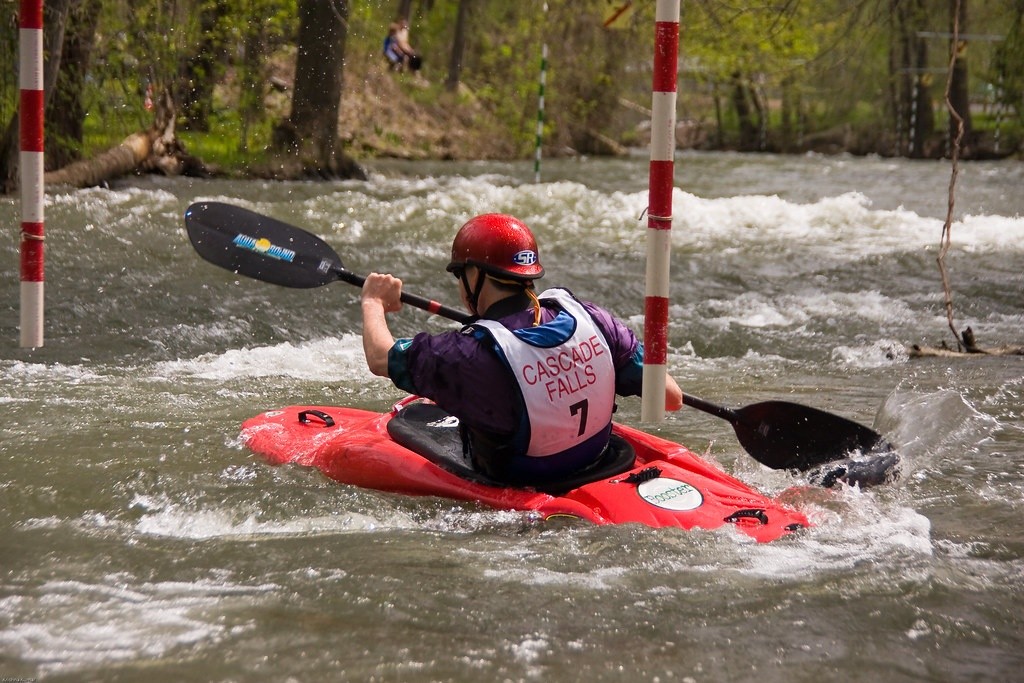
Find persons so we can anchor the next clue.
[360,213,685,476]
[382,22,407,73]
[394,16,421,77]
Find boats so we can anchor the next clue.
[241,396,814,542]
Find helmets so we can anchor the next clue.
[446,213,550,279]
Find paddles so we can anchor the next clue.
[180,199,900,488]
